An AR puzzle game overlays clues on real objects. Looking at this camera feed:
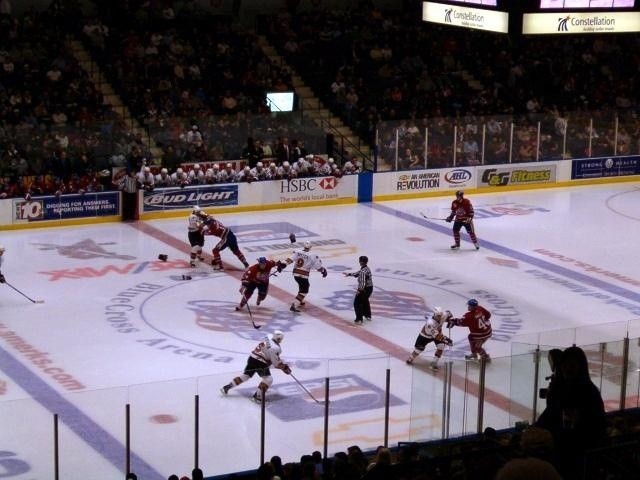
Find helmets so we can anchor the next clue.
[358,255,368,263]
[193,204,201,213]
[455,189,464,196]
[467,299,478,307]
[273,329,284,343]
[303,240,313,249]
[432,306,443,317]
[204,218,215,226]
[142,154,342,173]
[257,256,267,263]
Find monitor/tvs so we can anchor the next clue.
[265,91,295,112]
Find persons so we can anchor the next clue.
[444,297,494,363]
[446,189,480,250]
[0,248,7,284]
[188,205,207,267]
[234,257,287,313]
[406,305,454,370]
[223,329,291,403]
[341,255,375,323]
[532,346,608,479]
[195,212,249,269]
[277,239,328,312]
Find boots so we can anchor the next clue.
[252,388,262,402]
[223,382,233,395]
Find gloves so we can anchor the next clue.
[321,268,327,278]
[446,316,460,328]
[275,259,286,273]
[280,363,292,374]
[435,332,453,347]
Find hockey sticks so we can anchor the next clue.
[420,212,461,222]
[241,290,260,328]
[4,280,44,303]
[290,372,331,405]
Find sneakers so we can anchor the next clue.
[430,361,440,370]
[406,357,414,364]
[474,242,480,249]
[290,301,306,313]
[451,243,460,248]
[191,255,205,266]
[465,352,490,362]
[354,314,371,322]
[236,298,261,310]
